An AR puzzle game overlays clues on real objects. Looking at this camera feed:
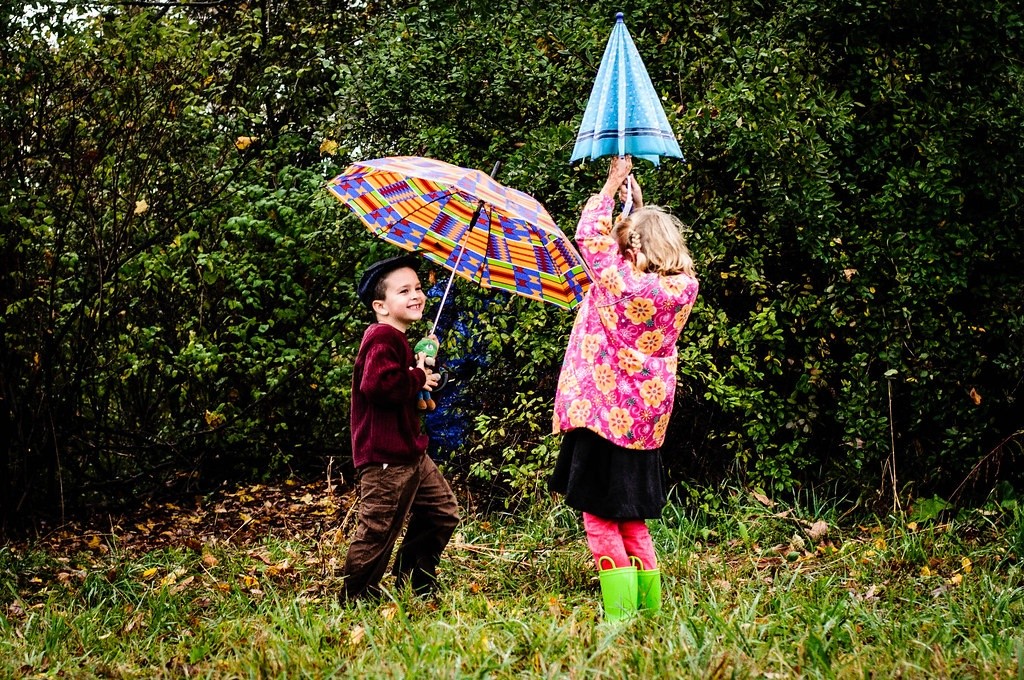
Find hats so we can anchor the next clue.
[356,255,422,307]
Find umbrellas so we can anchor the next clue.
[323,152,585,341]
[566,10,686,209]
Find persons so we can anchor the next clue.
[337,256,465,614]
[549,157,704,635]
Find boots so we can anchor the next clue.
[628,557,661,617]
[595,555,638,624]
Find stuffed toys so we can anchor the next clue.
[412,336,440,409]
